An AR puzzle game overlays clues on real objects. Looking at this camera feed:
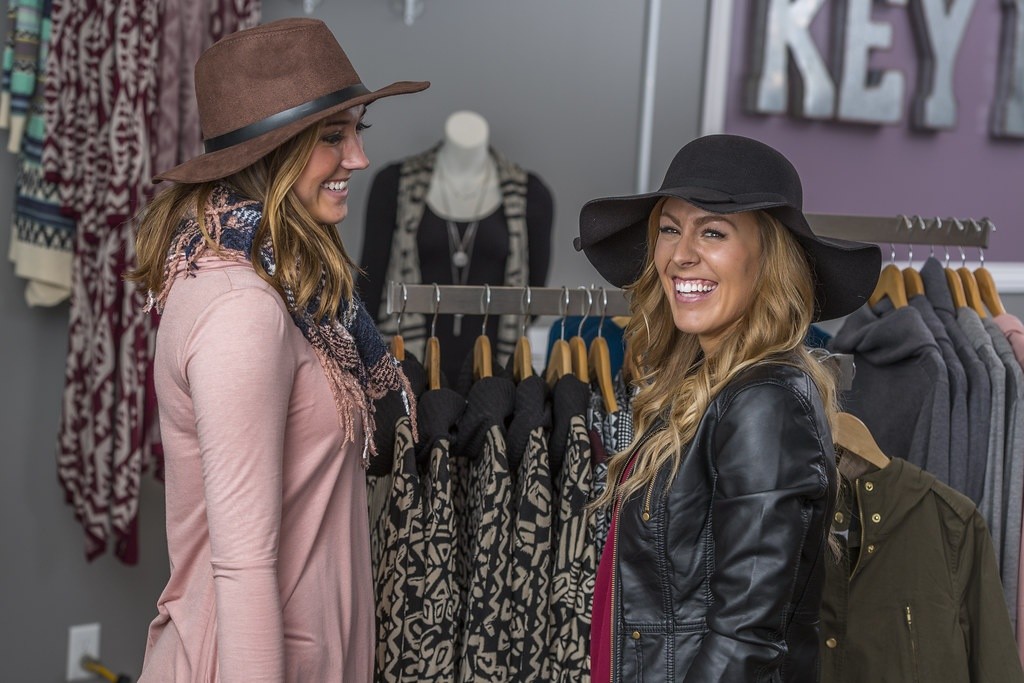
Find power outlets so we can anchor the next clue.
[66,623,101,681]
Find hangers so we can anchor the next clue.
[868,214,1006,318]
[807,353,891,469]
[392,282,632,416]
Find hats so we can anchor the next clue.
[150,17,431,185]
[574,134,883,323]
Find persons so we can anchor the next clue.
[126,18,430,683]
[573,134,881,683]
[356,111,555,378]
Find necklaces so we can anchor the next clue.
[436,164,490,337]
